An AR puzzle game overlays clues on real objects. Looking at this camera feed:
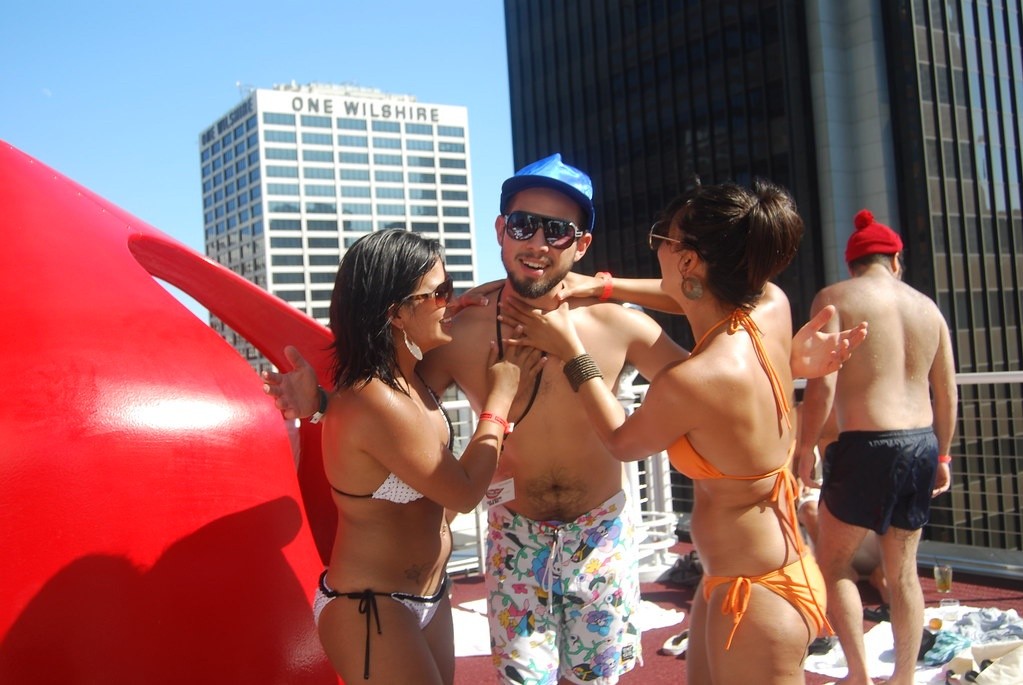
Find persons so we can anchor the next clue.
[797,207,958,685]
[311,227,549,685]
[499,173,826,685]
[262,154,867,685]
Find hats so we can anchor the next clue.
[845,209,904,263]
[501,153,595,234]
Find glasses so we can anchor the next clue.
[649,220,707,264]
[396,276,454,308]
[501,212,582,249]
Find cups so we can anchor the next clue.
[940,598,960,622]
[934,565,953,594]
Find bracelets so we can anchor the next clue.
[309,387,327,424]
[562,353,604,392]
[937,455,952,463]
[594,272,614,302]
[479,412,514,434]
